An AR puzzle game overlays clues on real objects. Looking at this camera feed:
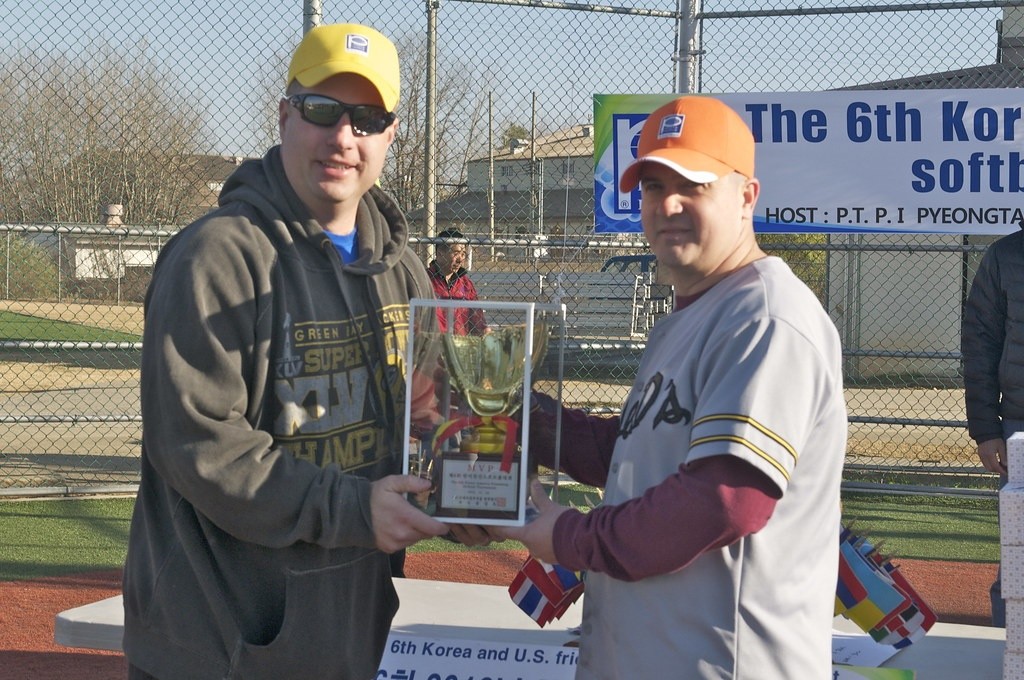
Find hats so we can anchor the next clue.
[620,95,754,194]
[285,22,400,112]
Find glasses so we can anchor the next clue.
[285,93,395,133]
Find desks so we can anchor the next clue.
[54,578,1006,680]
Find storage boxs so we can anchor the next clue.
[394,299,566,527]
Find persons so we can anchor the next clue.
[490,94,847,680]
[961,218,1023,627]
[425,227,488,339]
[121,23,503,680]
